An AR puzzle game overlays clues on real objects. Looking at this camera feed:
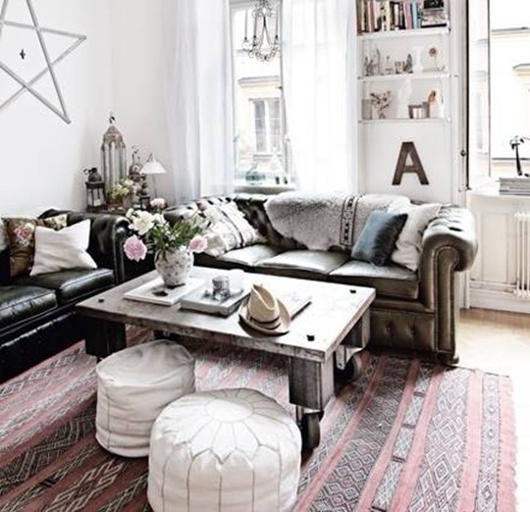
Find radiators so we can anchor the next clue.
[512,208,529,300]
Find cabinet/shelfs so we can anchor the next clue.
[352,1,459,122]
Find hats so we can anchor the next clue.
[237,284,291,335]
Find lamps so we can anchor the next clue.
[138,153,168,208]
[240,0,284,62]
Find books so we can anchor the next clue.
[356,0,420,34]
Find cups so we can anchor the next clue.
[408,105,423,118]
[360,98,373,120]
[212,275,229,301]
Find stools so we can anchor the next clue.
[91,339,303,512]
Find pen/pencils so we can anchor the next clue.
[291,301,311,321]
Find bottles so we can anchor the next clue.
[101,117,128,208]
[84,168,105,214]
[130,149,146,180]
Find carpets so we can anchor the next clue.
[0,324,514,512]
